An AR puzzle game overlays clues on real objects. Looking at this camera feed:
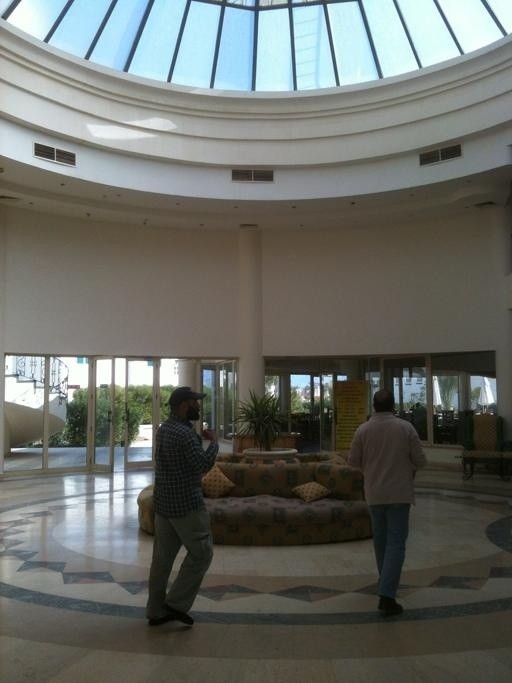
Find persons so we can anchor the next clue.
[346,386,429,617]
[143,385,222,633]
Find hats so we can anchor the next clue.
[169,387,207,403]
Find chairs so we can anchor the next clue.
[462,415,504,481]
[295,407,463,444]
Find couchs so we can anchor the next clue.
[139,450,374,544]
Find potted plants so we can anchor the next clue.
[232,388,301,465]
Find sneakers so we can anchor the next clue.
[159,603,194,625]
[148,614,174,625]
[378,597,403,613]
